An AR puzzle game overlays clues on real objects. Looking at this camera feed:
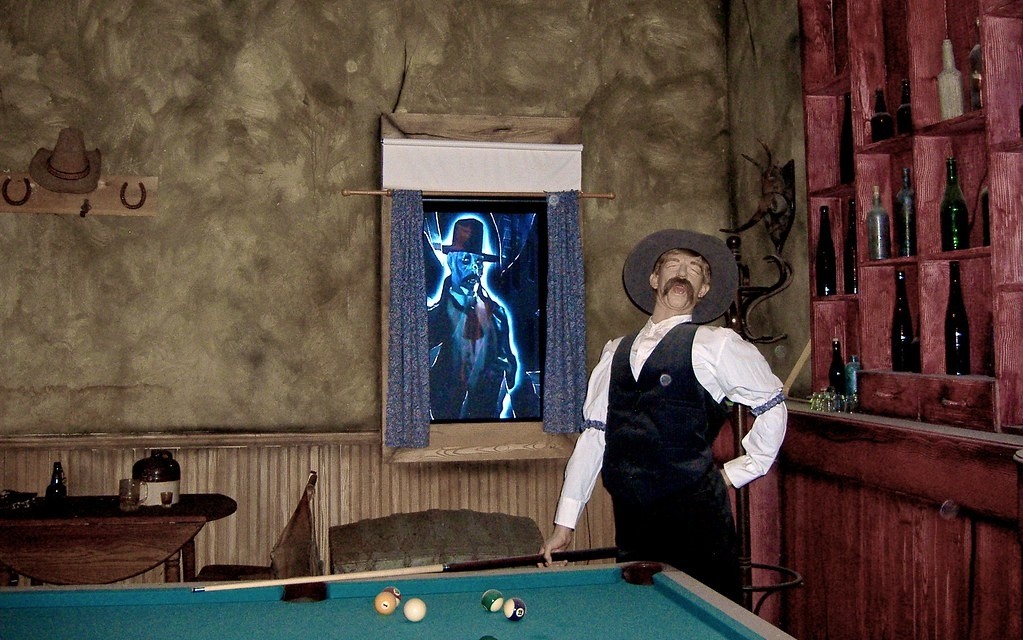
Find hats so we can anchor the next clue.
[28,128,100,193]
[622,228,739,323]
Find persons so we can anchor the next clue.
[538,227,790,608]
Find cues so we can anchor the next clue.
[189,543,622,593]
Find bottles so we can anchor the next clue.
[815,206,836,298]
[844,198,858,295]
[829,337,846,396]
[839,93,856,184]
[45,461,66,506]
[896,78,912,136]
[894,168,917,257]
[936,39,963,121]
[946,261,970,376]
[866,186,891,261]
[871,88,893,143]
[845,355,860,396]
[940,156,991,251]
[890,270,921,374]
[968,16,984,112]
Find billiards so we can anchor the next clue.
[373,585,529,622]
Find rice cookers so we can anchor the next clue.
[132,449,181,507]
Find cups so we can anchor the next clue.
[119,479,148,511]
[161,492,173,509]
[809,390,861,416]
